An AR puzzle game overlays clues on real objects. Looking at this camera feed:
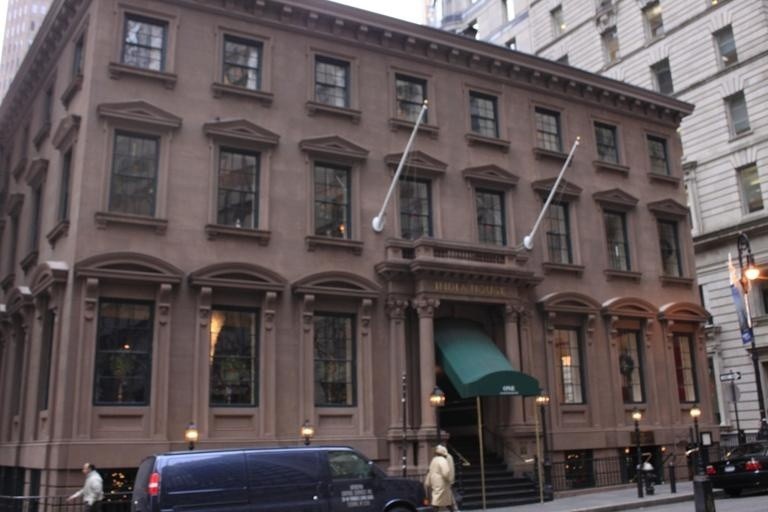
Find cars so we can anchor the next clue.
[705,440,768,497]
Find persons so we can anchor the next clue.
[65,463,105,512]
[424,445,456,512]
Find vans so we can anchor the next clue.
[130,444,425,511]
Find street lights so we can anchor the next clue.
[736,232,767,440]
[631,406,645,498]
[302,419,315,446]
[536,388,554,501]
[185,421,197,450]
[689,403,701,446]
[429,385,447,443]
